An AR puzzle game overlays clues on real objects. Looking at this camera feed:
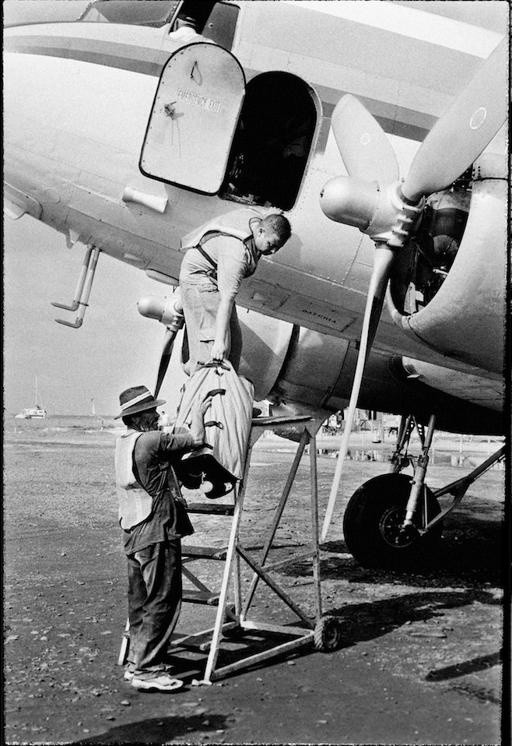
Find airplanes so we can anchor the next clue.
[0,0,509,571]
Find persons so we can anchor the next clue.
[176,205,295,418]
[111,384,215,694]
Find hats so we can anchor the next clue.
[113,385,167,421]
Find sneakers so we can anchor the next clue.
[123,662,185,694]
